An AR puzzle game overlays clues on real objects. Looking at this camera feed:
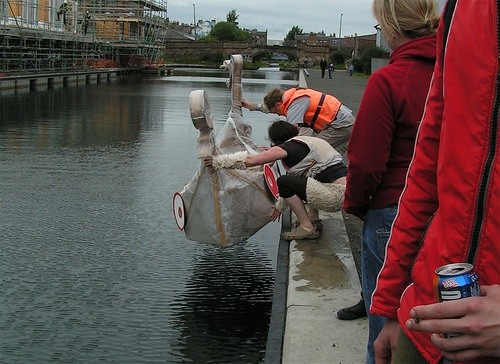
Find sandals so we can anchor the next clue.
[282,225,320,240]
[294,219,323,230]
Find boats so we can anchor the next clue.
[172,54,281,248]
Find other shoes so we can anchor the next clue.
[336,291,368,321]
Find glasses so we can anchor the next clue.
[374,23,381,34]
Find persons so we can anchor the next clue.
[320,57,327,78]
[344,0,440,364]
[369,0,500,364]
[349,63,354,76]
[328,60,335,71]
[203,120,348,240]
[241,88,355,152]
[56,0,68,24]
[80,11,91,36]
[328,64,333,79]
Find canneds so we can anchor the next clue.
[432,262,483,340]
